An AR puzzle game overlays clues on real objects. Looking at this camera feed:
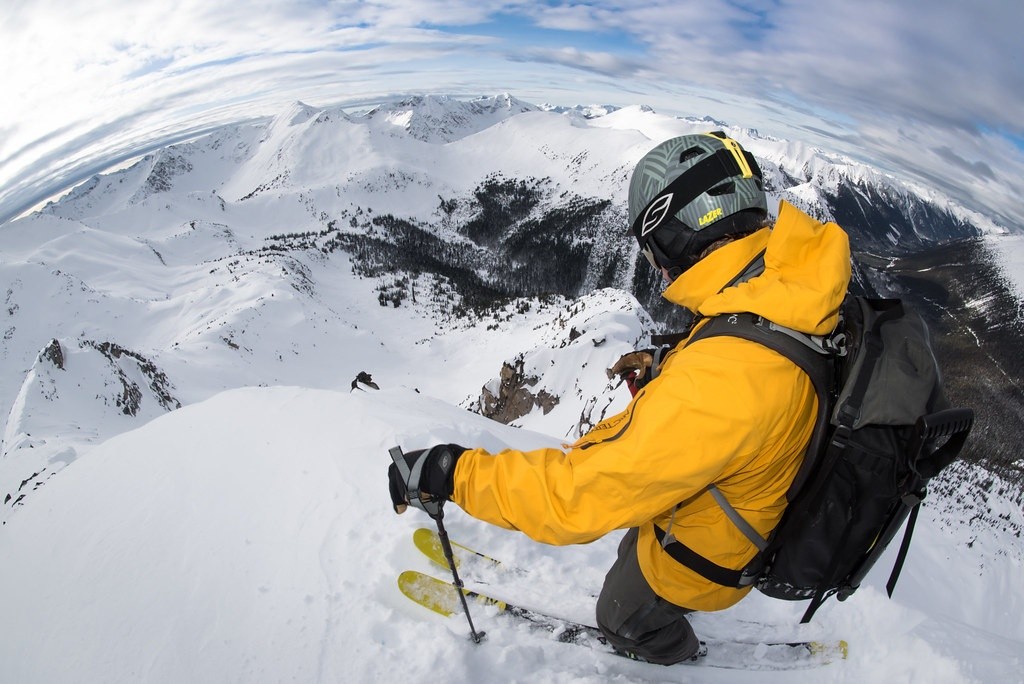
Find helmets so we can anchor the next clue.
[626,132,768,271]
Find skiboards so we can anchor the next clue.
[397,527,849,672]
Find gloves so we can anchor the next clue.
[388,446,473,514]
[608,350,656,389]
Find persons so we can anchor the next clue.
[388,130,854,674]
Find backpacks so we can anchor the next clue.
[651,294,976,625]
